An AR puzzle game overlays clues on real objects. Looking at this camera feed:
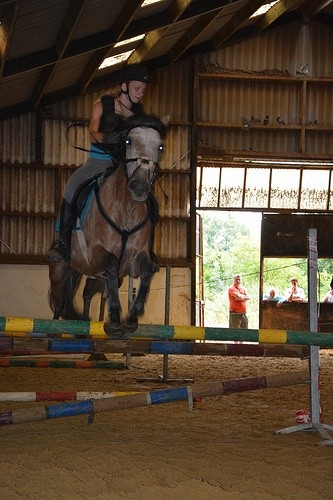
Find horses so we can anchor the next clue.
[47,99,174,337]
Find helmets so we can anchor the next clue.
[120,64,149,83]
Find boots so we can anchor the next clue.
[149,223,158,264]
[48,198,77,259]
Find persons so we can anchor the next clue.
[263,290,286,307]
[48,64,161,274]
[228,274,250,344]
[323,282,333,302]
[285,278,305,303]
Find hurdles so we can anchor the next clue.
[0,229,333,442]
